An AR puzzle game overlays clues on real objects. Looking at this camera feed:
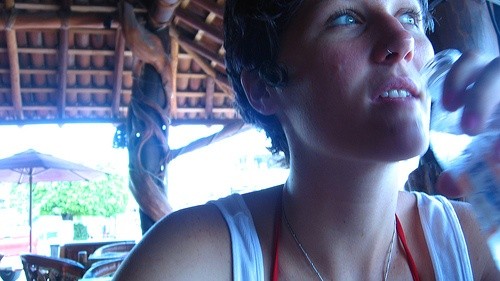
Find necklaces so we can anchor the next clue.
[281,176,398,281]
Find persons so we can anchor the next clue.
[113,1,499,281]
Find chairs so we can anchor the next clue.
[21,241,133,280]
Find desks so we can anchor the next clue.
[88,252,131,270]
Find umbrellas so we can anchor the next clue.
[0,147,112,255]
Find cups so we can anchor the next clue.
[0,255,23,281]
[50,245,59,258]
